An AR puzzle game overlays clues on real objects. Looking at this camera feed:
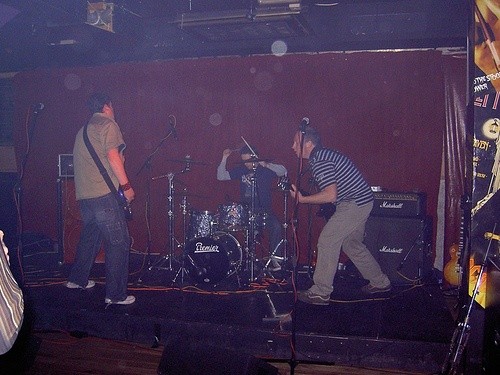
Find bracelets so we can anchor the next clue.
[121,182,131,191]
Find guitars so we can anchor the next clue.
[115,187,135,220]
[275,175,336,221]
[443,194,466,287]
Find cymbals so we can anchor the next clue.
[164,158,214,167]
[234,157,274,163]
[159,192,218,200]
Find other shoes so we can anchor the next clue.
[105,296,136,304]
[66,280,96,289]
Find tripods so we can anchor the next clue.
[236,163,294,291]
[259,134,335,375]
[138,163,201,287]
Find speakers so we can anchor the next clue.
[157,339,279,375]
[361,216,434,287]
[0,329,43,375]
[56,178,106,266]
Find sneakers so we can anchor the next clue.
[297,291,329,305]
[361,284,391,294]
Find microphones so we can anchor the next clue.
[30,103,45,109]
[396,260,405,272]
[301,118,309,133]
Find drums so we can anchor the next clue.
[220,204,250,229]
[182,231,243,289]
[244,207,273,227]
[187,209,220,240]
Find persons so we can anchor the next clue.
[0,230,24,355]
[66,94,136,305]
[288,127,396,306]
[216,145,288,256]
